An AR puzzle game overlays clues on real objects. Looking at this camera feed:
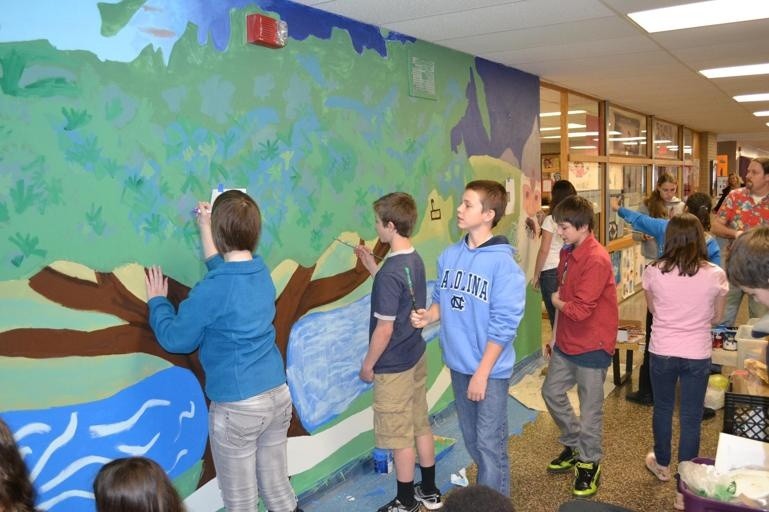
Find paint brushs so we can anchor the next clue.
[334,237,383,261]
[536,213,547,217]
[404,267,417,314]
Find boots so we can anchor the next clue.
[627,366,653,405]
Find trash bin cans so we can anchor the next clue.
[676,457,769,512]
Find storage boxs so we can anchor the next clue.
[735,325,768,370]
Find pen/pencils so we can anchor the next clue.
[193,209,212,215]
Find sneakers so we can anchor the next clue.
[674,491,685,510]
[378,498,420,512]
[572,458,600,498]
[647,453,670,481]
[413,481,443,510]
[547,446,580,472]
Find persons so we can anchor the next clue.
[0,417,37,512]
[642,212,728,483]
[530,180,577,376]
[541,195,618,496]
[355,192,444,511]
[409,180,527,499]
[144,190,302,512]
[93,456,185,512]
[610,156,768,419]
[439,485,516,512]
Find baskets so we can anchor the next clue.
[724,377,769,442]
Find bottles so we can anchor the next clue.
[712,334,737,351]
